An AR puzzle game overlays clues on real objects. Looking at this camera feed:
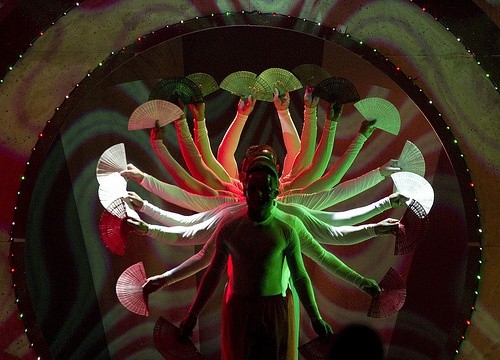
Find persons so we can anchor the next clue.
[121,88,411,360]
[177,163,333,359]
